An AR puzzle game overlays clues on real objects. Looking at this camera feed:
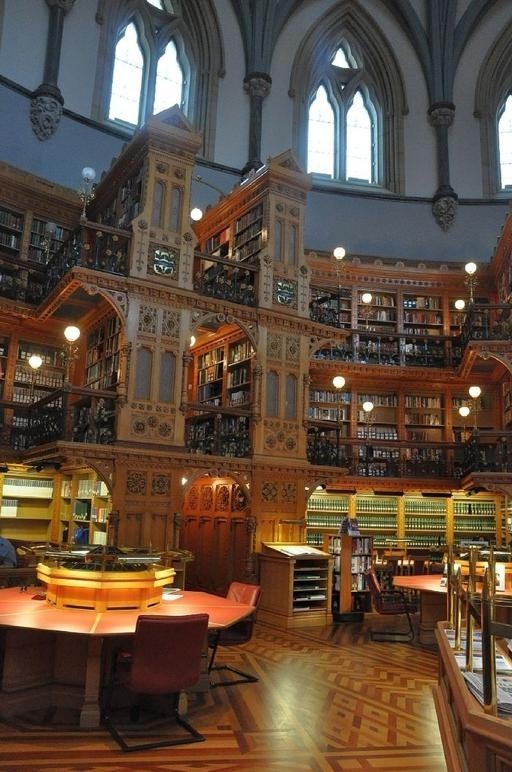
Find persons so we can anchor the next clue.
[0,535,20,588]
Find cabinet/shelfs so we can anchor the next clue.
[322,533,373,615]
[1,156,512,566]
[255,541,337,628]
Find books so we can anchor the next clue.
[204,205,262,280]
[309,376,511,477]
[188,340,255,458]
[326,291,489,356]
[497,263,512,306]
[1,315,121,451]
[444,628,512,717]
[1,479,109,545]
[0,176,138,265]
[307,498,511,561]
[280,536,371,613]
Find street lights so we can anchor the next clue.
[465,262,476,303]
[456,299,465,333]
[470,387,481,427]
[29,356,43,405]
[83,168,96,216]
[333,248,345,287]
[362,294,372,325]
[363,401,373,438]
[459,407,470,440]
[64,326,80,383]
[44,223,55,263]
[333,376,346,421]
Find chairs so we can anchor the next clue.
[102,613,210,753]
[208,582,264,688]
[366,568,417,643]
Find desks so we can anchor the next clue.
[392,574,512,645]
[0,585,256,727]
